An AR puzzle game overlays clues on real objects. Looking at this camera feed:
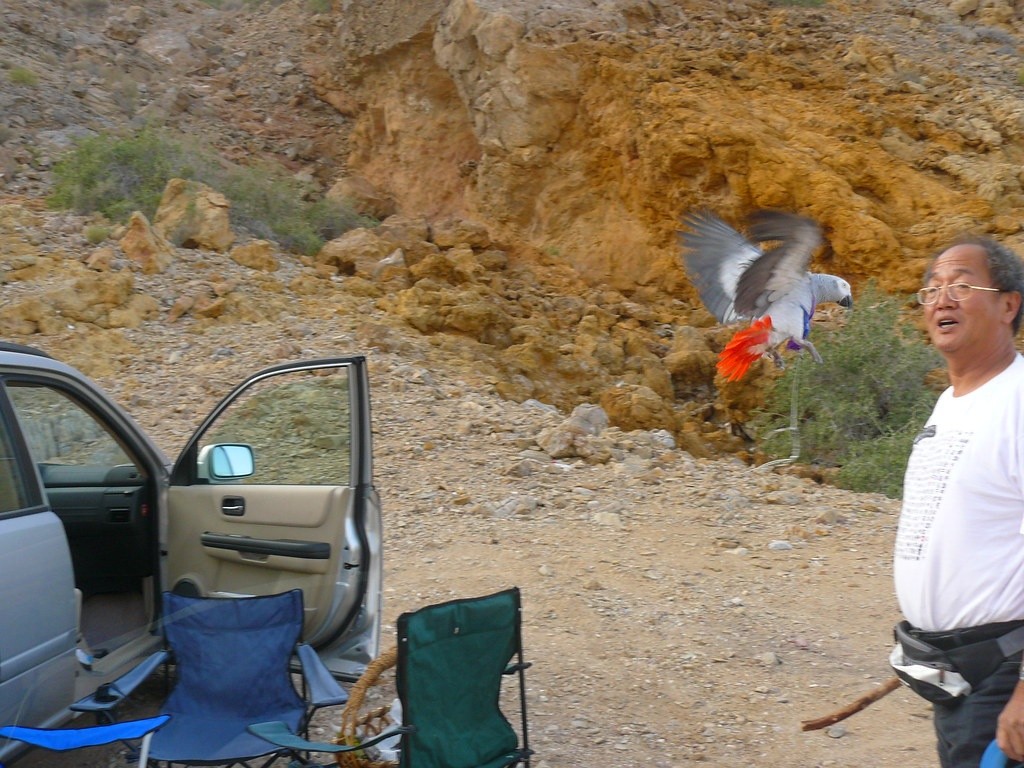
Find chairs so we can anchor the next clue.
[66,588,350,768]
[245,586,535,768]
[0,714,172,768]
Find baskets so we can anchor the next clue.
[331,647,400,768]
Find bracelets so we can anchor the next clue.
[1018,659,1024,681]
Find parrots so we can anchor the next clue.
[672,202,854,373]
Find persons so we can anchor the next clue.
[893,235,1024,767]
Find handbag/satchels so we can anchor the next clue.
[888,621,1024,704]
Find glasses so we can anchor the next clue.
[917,282,1011,306]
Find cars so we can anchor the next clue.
[0,333,387,768]
